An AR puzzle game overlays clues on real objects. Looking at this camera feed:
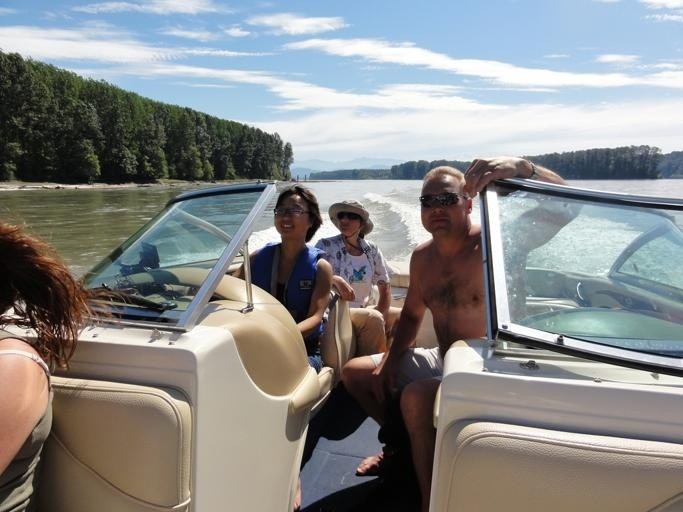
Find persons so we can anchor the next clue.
[1,220,127,512]
[340,155,578,511]
[314,198,393,390]
[232,184,333,511]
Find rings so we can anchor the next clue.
[469,172,475,177]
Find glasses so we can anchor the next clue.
[419,192,469,209]
[274,207,311,217]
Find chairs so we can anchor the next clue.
[307,285,342,420]
[334,288,358,367]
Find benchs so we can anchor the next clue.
[24,373,193,511]
[433,419,683,512]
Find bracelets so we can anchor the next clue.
[529,161,540,180]
